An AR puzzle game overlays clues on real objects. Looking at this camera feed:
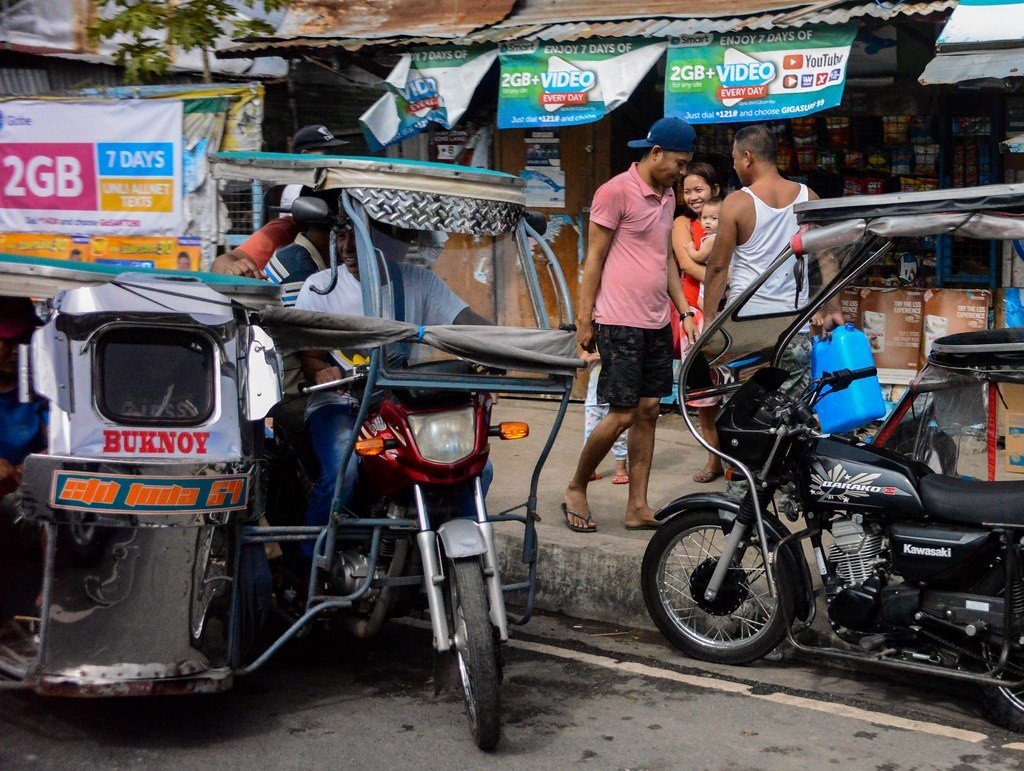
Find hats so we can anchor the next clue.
[628,117,696,152]
[291,125,350,152]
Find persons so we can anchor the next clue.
[579,343,630,484]
[682,196,733,314]
[668,163,727,485]
[0,125,495,640]
[560,116,700,532]
[702,126,845,401]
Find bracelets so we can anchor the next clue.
[679,311,695,320]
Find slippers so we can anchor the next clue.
[589,474,602,481]
[626,511,671,530]
[694,469,724,483]
[561,503,596,532]
[612,474,630,484]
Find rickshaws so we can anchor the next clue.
[637,182,1024,737]
[0,151,592,754]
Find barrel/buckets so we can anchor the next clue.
[806,323,886,435]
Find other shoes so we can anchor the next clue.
[0,628,39,661]
[289,594,338,622]
